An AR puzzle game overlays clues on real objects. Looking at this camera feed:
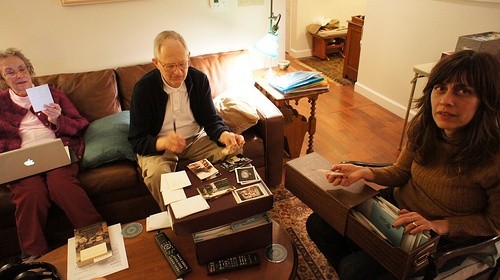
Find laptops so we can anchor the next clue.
[0,139,71,185]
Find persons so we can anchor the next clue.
[129,31,245,213]
[305,49,500,280]
[0,48,101,262]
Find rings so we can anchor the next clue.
[412,221,418,226]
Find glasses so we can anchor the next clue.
[1,67,30,77]
[156,58,191,71]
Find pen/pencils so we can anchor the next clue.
[173,121,176,133]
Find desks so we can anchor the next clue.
[399,59,438,153]
[259,50,329,156]
[312,26,348,60]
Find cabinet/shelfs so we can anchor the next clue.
[343,20,363,83]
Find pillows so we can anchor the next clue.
[79,109,134,165]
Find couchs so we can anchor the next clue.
[0,51,288,246]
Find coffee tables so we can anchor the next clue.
[37,211,295,280]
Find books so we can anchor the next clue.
[74,222,113,268]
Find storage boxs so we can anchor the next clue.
[454,31,500,55]
[161,158,273,264]
[284,153,439,278]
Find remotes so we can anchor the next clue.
[206,252,260,275]
[154,232,192,278]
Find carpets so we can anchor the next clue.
[296,55,354,87]
